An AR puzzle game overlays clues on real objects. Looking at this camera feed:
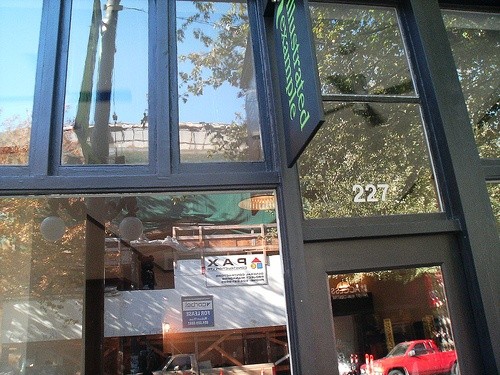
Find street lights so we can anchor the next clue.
[38,196,144,375]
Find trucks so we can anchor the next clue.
[152,353,276,375]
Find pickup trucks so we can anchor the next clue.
[359,339,457,375]
